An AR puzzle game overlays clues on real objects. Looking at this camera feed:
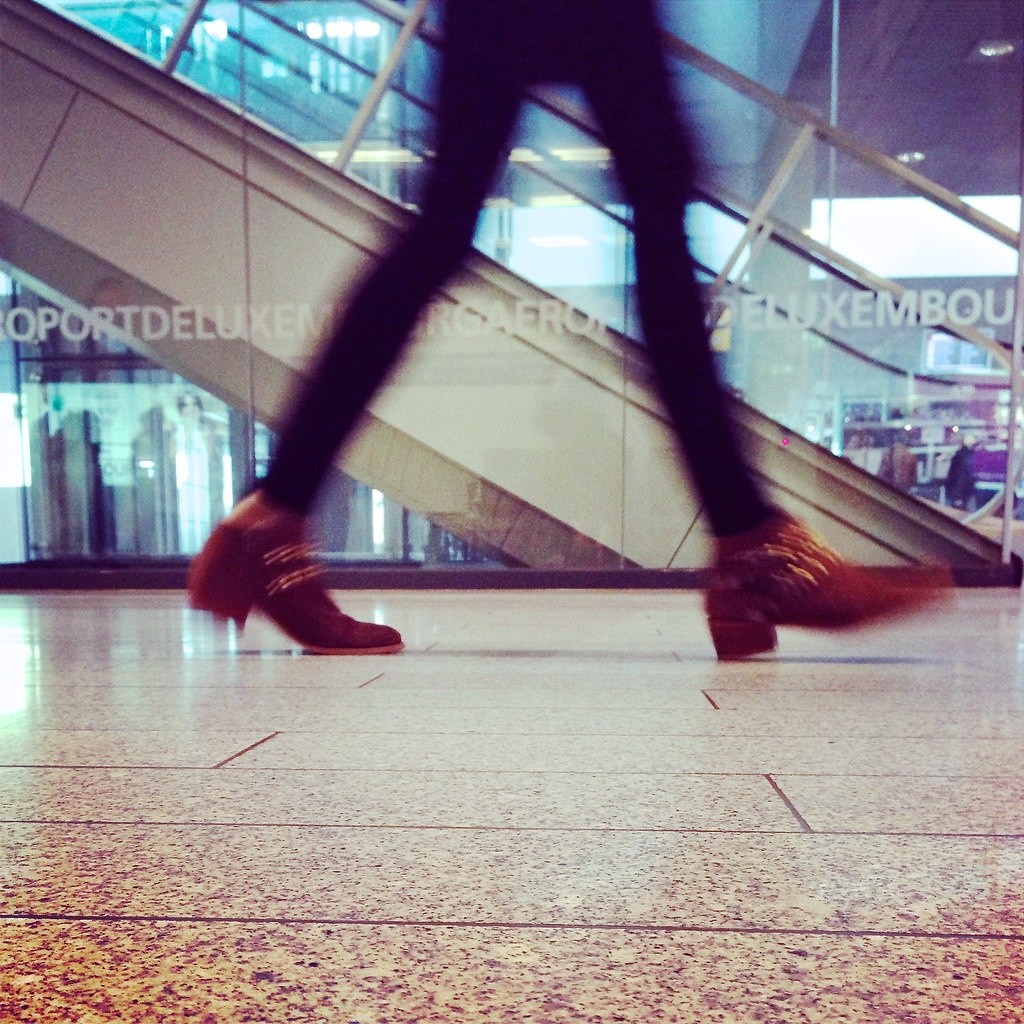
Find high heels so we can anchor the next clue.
[707,507,945,658]
[188,489,405,653]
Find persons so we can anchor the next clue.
[946,433,978,510]
[882,434,919,491]
[189,0,951,660]
